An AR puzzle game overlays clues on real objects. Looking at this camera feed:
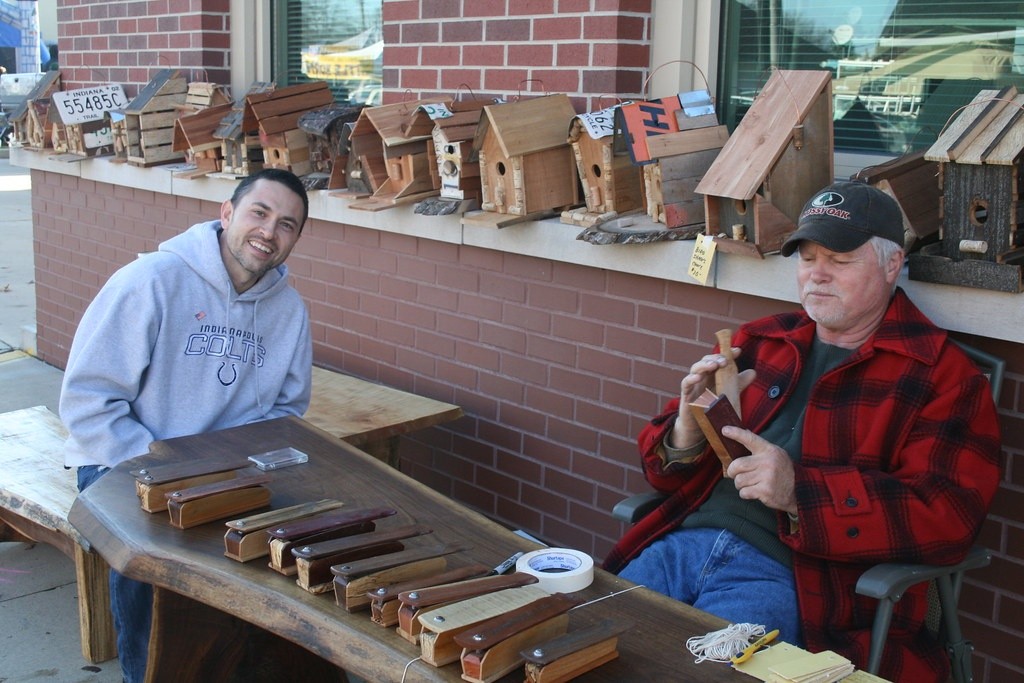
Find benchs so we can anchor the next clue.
[0,405,117,664]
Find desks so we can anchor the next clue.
[67,415,888,683]
[302,366,464,471]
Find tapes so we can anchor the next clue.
[515,547,594,593]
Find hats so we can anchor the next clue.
[779,179,905,257]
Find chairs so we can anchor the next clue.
[610,337,1005,683]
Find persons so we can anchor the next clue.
[603,178,1001,683]
[60,168,312,683]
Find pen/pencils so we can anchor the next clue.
[727,629,779,666]
[486,552,523,576]
